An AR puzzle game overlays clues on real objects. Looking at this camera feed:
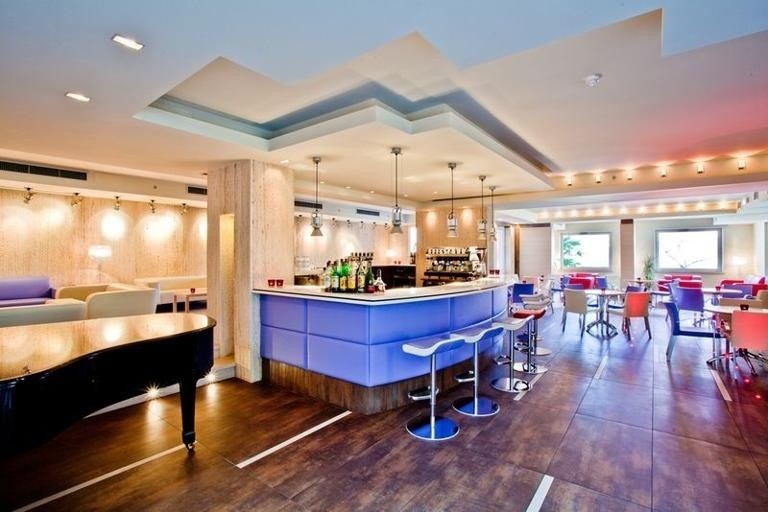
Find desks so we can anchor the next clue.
[173,288,207,313]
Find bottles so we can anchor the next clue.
[601,288,605,294]
[348,258,357,293]
[340,262,347,293]
[357,262,366,293]
[324,274,331,292]
[427,248,465,254]
[351,252,374,266]
[331,261,340,292]
[339,259,344,276]
[409,252,415,264]
[373,269,387,294]
[322,267,328,290]
[365,267,373,293]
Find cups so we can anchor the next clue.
[277,280,284,286]
[190,288,195,293]
[268,280,275,287]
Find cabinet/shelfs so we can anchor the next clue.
[420,248,487,287]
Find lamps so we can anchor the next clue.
[478,176,486,240]
[311,157,324,236]
[563,159,747,187]
[23,187,191,216]
[488,186,496,243]
[447,163,456,238]
[390,148,403,234]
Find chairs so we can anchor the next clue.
[0,276,158,327]
[558,273,654,342]
[449,324,504,417]
[400,336,466,440]
[509,275,553,375]
[730,311,768,381]
[663,301,723,366]
[655,274,768,309]
[490,315,533,393]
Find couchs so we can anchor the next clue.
[134,275,207,304]
